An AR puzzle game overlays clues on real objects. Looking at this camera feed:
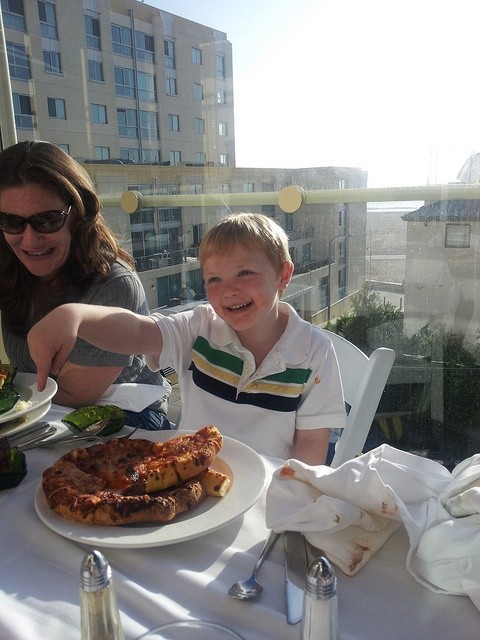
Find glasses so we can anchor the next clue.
[1,204,75,233]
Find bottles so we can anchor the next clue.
[302,557,340,640]
[80,549,122,639]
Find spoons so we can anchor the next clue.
[18,420,109,452]
[226,530,281,600]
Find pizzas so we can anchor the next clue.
[42,424,229,525]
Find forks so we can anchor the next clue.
[39,426,139,448]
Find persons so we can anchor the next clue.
[29,212,349,468]
[1,138,172,432]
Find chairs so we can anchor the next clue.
[339,319,454,461]
[319,328,397,471]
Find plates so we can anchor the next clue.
[0,372,58,439]
[34,429,267,549]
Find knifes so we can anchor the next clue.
[283,531,310,625]
